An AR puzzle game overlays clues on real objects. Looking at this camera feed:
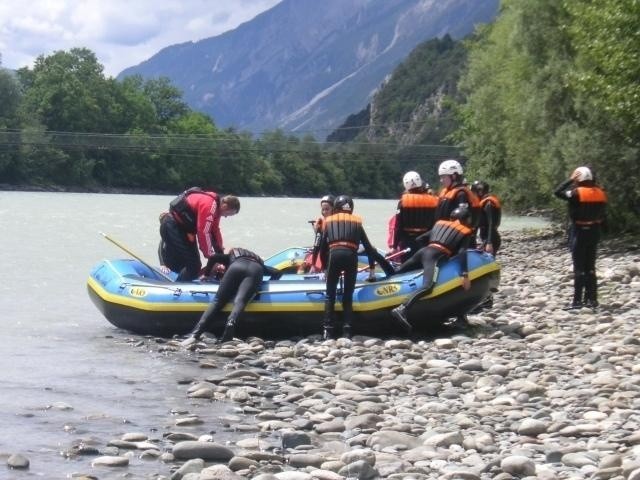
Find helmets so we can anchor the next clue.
[438,160,463,176]
[321,195,335,206]
[450,208,473,226]
[333,195,354,213]
[403,172,423,190]
[572,166,593,182]
[471,180,489,197]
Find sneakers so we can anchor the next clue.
[182,329,201,338]
[561,298,599,311]
[340,324,352,340]
[321,325,334,340]
[448,319,468,329]
[220,322,235,342]
[390,303,414,332]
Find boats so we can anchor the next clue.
[85,247,501,334]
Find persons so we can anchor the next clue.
[158,186,241,285]
[295,192,338,275]
[552,164,609,311]
[308,194,377,341]
[156,208,203,277]
[180,245,283,343]
[386,158,504,332]
